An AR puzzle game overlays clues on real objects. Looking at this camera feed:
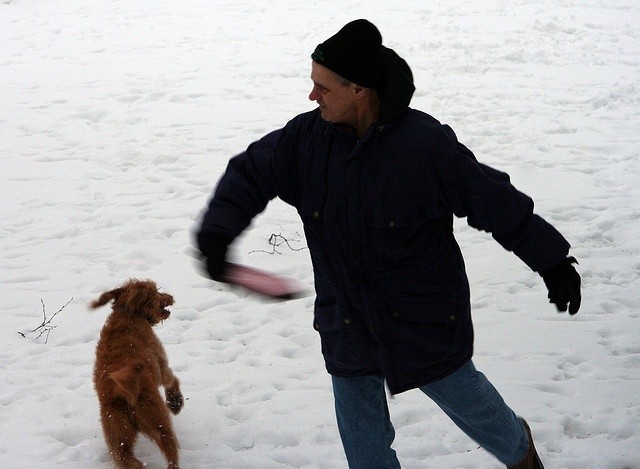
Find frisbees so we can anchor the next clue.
[224,262,313,299]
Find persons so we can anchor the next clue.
[196,19,581,469]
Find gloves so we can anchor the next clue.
[538,253,581,314]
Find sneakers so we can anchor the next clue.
[506,420,544,469]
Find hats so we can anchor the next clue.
[312,20,381,89]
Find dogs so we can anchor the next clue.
[90,278,184,469]
